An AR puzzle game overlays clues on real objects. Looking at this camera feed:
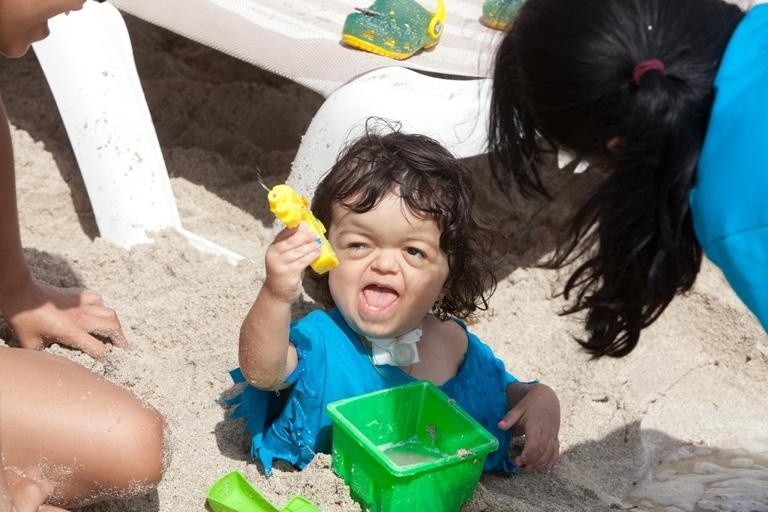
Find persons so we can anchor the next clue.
[1,1,172,512]
[216,117,560,480]
[481,0,768,357]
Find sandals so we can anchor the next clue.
[482,1,525,31]
[344,1,444,60]
